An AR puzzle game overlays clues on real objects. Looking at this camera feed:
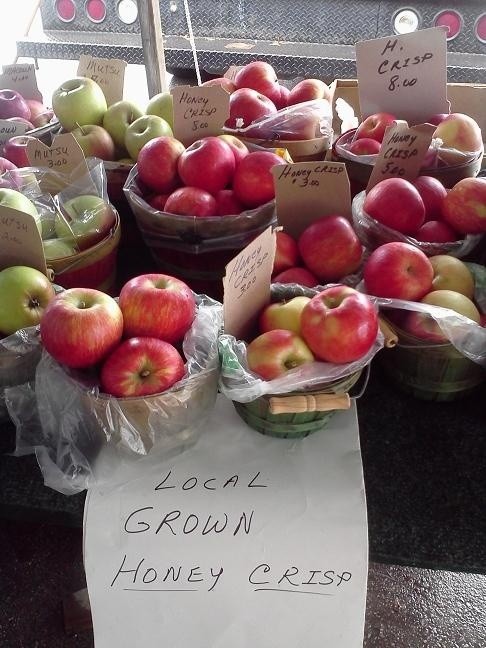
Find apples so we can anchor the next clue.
[53,77,174,164]
[42,272,194,397]
[2,136,34,167]
[137,136,288,216]
[363,243,486,343]
[0,156,115,262]
[362,177,486,243]
[198,61,330,141]
[270,214,366,285]
[350,113,481,167]
[248,286,378,379]
[0,267,57,335]
[0,89,54,129]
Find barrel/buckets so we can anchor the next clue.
[219,283,373,437]
[1,281,64,422]
[45,284,219,461]
[357,261,485,406]
[125,141,278,301]
[45,199,120,297]
[333,127,485,202]
[55,125,141,199]
[349,181,483,260]
[220,122,334,162]
